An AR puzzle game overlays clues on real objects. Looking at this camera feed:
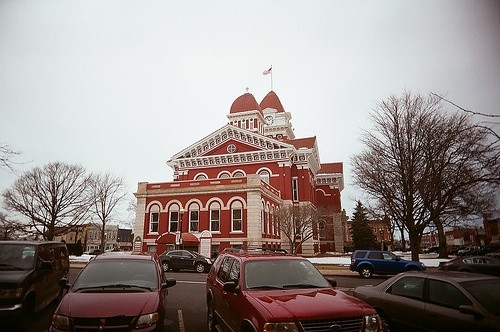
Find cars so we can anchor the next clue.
[0,240,71,327]
[152,250,213,273]
[49,250,176,332]
[421,239,500,258]
[354,270,500,332]
[438,255,500,277]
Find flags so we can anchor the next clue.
[262,67,271,75]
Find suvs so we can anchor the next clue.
[350,250,425,278]
[205,244,390,332]
